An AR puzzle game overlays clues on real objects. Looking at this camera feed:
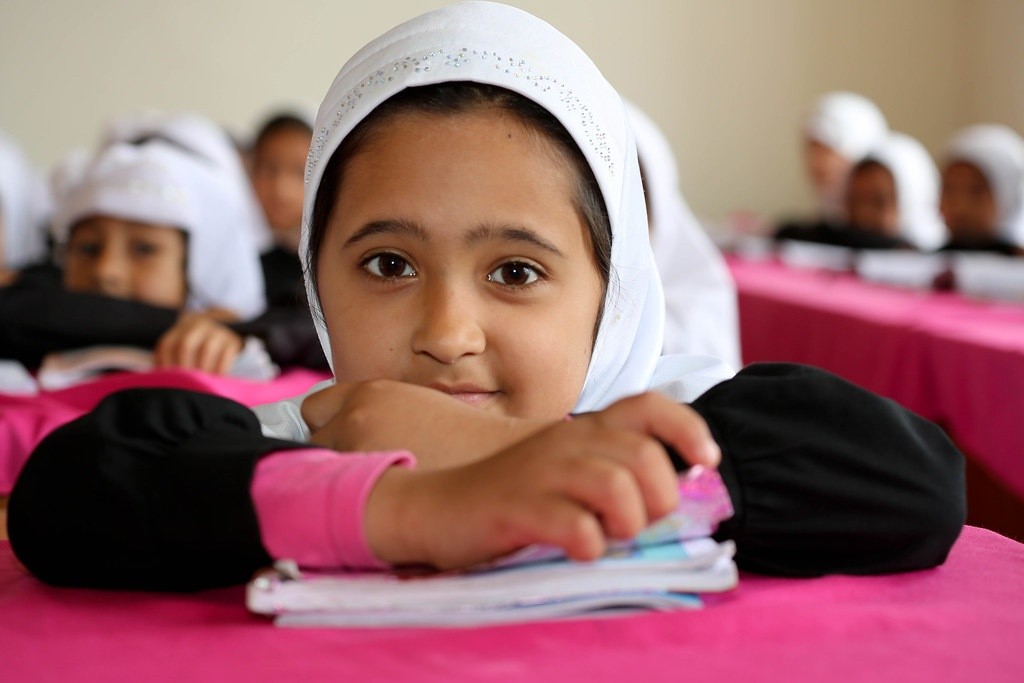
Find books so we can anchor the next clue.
[247,464,739,632]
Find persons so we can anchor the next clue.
[742,96,1024,308]
[6,1,968,593]
[0,111,328,385]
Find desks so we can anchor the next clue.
[0,251,1023,683]
